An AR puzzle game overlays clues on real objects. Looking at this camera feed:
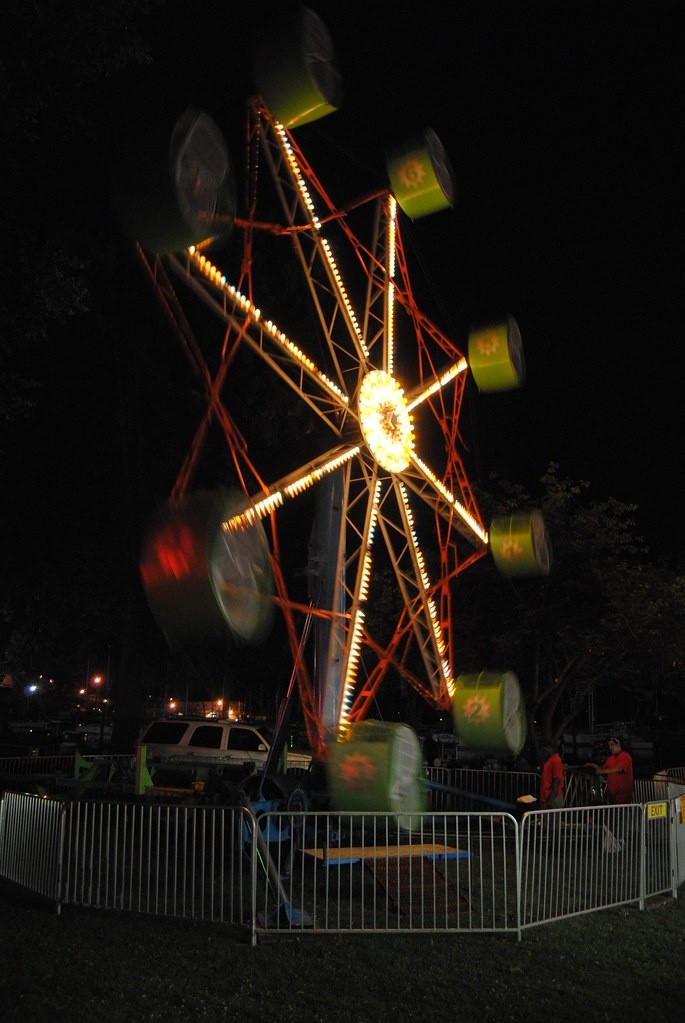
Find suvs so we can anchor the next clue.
[134,716,312,785]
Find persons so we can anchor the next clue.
[538,737,565,854]
[586,735,635,848]
